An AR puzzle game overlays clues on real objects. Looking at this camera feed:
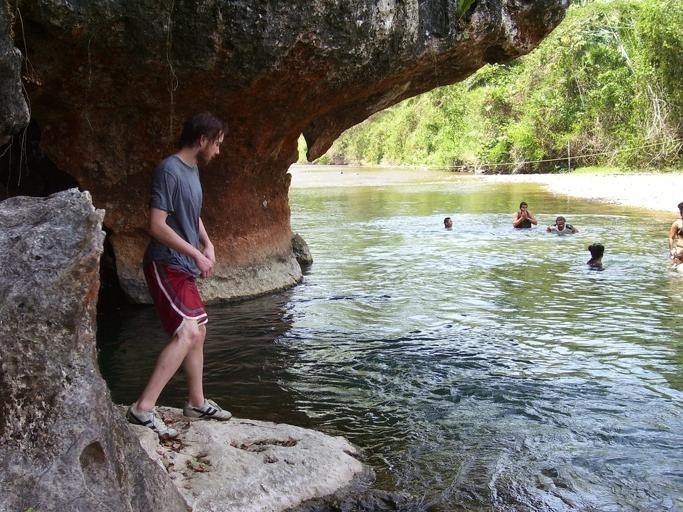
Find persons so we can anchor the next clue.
[444,217,453,228]
[586,243,604,267]
[669,202,683,274]
[512,202,579,235]
[126,112,233,439]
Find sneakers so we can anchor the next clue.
[183,398,232,421]
[125,402,180,441]
